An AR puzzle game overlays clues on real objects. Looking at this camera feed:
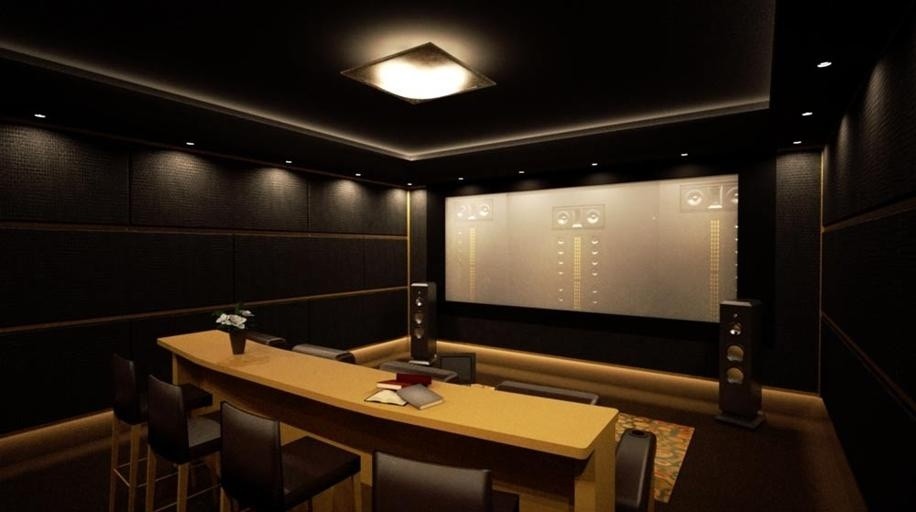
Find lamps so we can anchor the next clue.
[338,42,500,104]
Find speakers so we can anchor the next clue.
[718,299,763,420]
[410,283,437,361]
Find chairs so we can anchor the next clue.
[107,355,213,512]
[217,400,363,512]
[246,330,287,347]
[495,378,600,406]
[146,373,222,512]
[615,429,656,509]
[372,450,520,512]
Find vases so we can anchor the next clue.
[228,326,247,354]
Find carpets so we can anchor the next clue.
[472,382,695,503]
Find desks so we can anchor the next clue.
[158,328,620,511]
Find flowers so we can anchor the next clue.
[210,301,255,329]
